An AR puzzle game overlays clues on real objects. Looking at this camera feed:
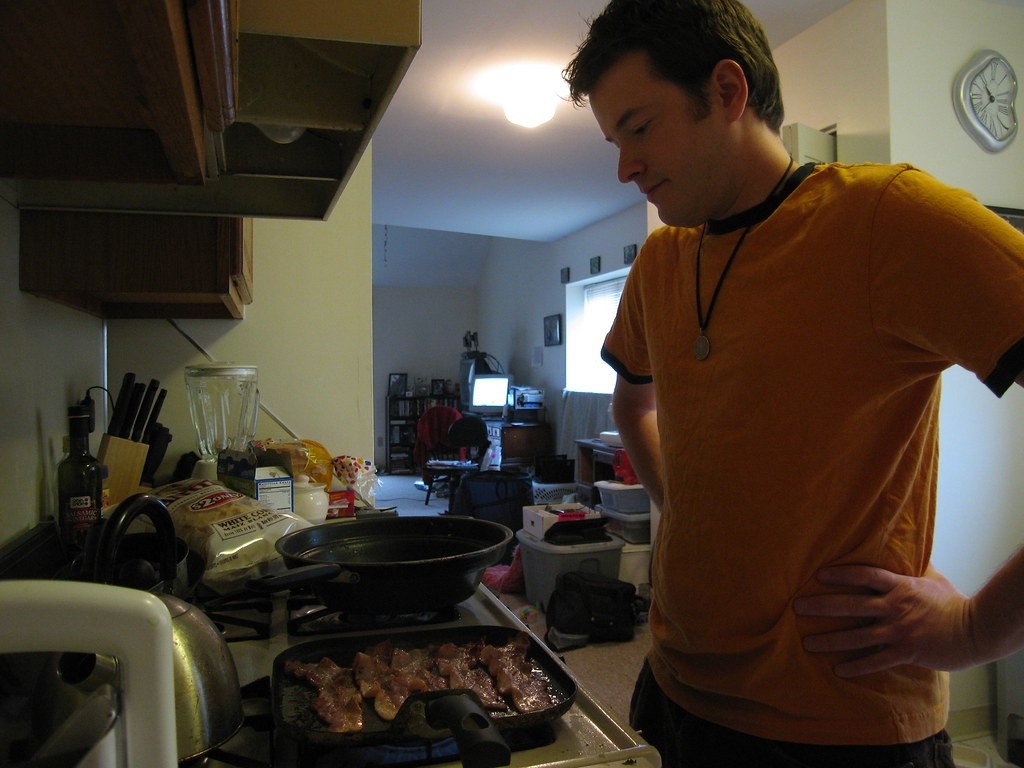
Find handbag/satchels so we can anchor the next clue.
[541,559,638,652]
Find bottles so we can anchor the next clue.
[58,421,102,551]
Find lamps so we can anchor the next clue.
[501,92,556,129]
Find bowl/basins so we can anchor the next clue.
[275,515,512,611]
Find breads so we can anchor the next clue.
[247,440,333,491]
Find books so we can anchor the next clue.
[390,397,456,474]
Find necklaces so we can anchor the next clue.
[694,157,792,360]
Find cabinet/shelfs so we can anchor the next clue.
[386,396,461,474]
[0,0,423,220]
[20,209,254,319]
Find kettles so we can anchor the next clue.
[58,492,243,762]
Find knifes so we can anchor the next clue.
[106,373,168,443]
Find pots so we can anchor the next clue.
[272,625,578,768]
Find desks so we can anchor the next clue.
[485,421,549,472]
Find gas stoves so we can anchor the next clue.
[197,574,557,768]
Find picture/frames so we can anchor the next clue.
[543,314,561,347]
[432,379,445,397]
[624,244,637,264]
[388,373,407,396]
[590,256,600,273]
[560,267,569,283]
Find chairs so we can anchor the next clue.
[418,407,488,510]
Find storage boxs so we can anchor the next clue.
[516,527,625,612]
[608,533,651,594]
[595,481,650,542]
[216,444,294,511]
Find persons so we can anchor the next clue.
[565,0,1024,768]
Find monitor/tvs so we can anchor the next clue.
[459,357,515,417]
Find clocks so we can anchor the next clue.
[952,50,1018,153]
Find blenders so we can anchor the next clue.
[184,363,259,483]
